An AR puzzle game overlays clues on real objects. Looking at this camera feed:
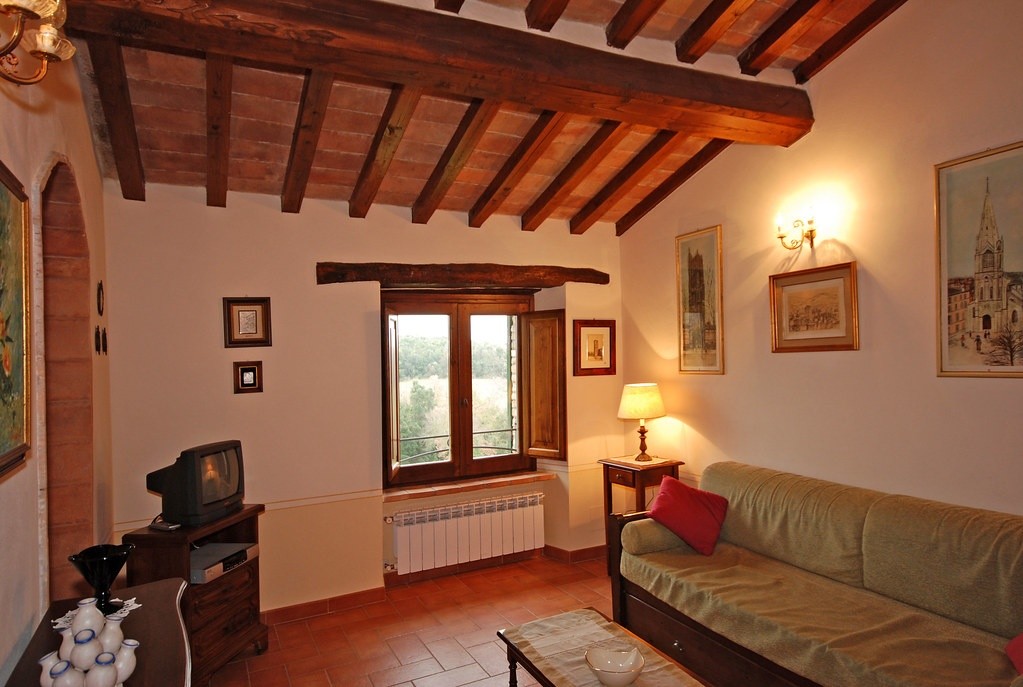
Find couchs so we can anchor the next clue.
[618,461,1023,687]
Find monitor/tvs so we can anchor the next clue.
[146,440,244,529]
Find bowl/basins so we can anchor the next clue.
[585,640,645,686]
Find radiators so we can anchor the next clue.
[394,491,546,578]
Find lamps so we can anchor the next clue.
[616,383,666,462]
[0,0,77,85]
[776,209,815,252]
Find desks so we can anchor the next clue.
[597,454,685,576]
[3,576,191,687]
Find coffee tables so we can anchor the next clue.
[496,606,716,687]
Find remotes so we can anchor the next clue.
[148,522,181,530]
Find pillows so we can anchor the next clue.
[644,475,728,556]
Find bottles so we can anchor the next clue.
[37,597,139,687]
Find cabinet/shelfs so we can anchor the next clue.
[122,503,268,687]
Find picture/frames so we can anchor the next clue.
[573,319,617,376]
[0,161,32,482]
[223,297,272,349]
[768,261,860,353]
[673,224,725,374]
[933,140,1023,378]
[233,361,263,394]
